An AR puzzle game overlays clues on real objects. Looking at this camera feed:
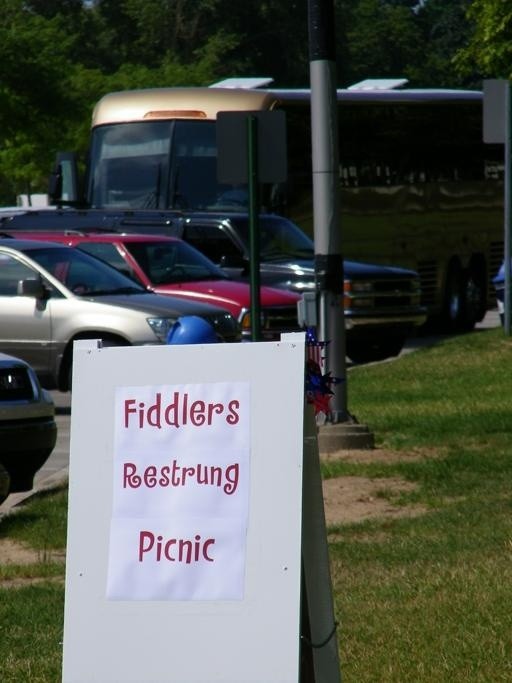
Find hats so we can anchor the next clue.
[0,350,58,510]
[0,239,244,403]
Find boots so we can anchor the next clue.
[0,206,431,365]
[1,229,303,341]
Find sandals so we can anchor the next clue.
[54,77,504,332]
